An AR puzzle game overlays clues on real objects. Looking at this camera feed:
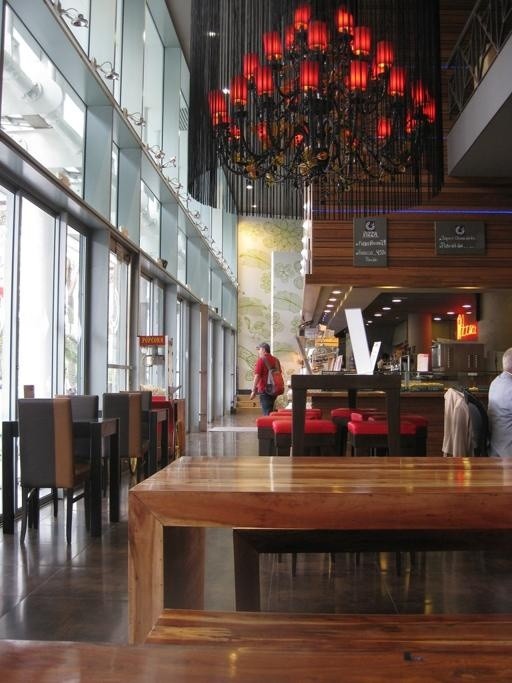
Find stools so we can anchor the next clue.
[256,408,427,581]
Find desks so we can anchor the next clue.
[291,375,401,576]
[128,456,512,651]
[2,417,120,538]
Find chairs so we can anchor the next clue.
[18,390,186,544]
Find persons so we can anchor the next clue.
[485,347,512,457]
[377,355,387,371]
[250,343,282,417]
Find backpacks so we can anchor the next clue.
[258,355,284,395]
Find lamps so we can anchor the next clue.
[188,0,442,218]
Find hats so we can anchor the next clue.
[255,342,270,351]
